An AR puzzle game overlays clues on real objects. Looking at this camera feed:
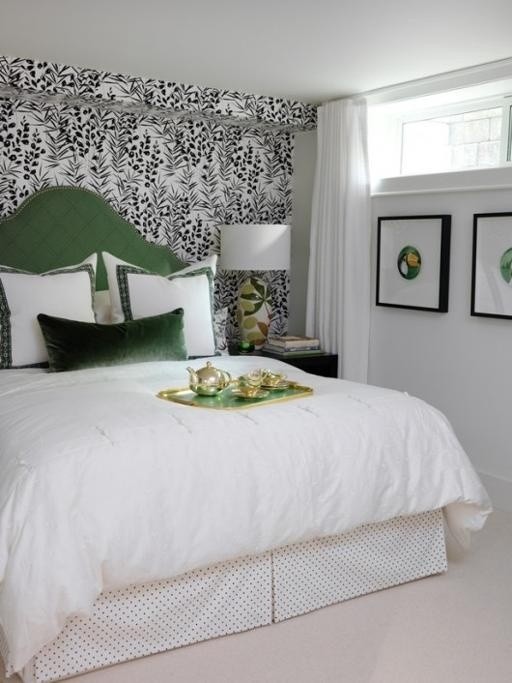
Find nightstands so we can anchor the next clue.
[228,346,338,378]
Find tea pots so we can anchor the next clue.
[185,361,232,396]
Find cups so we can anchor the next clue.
[237,369,283,396]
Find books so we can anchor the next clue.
[261,347,325,355]
[264,343,320,351]
[268,335,319,347]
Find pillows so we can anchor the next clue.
[0,249,218,374]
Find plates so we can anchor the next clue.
[232,384,288,401]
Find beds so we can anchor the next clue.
[1,184,451,683]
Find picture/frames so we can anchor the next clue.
[374,211,512,319]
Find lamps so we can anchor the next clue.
[219,222,291,350]
